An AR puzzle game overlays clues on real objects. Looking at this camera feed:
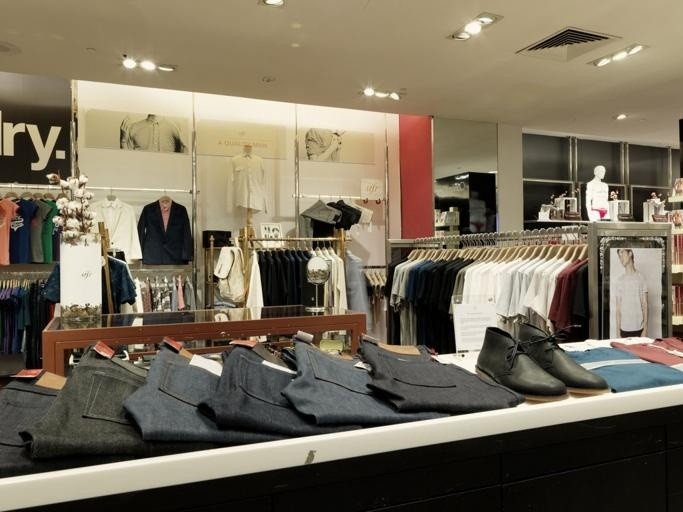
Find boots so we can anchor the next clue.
[476,326,568,401]
[517,322,611,395]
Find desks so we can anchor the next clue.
[43,302,368,378]
[1,333,683,512]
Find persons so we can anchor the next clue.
[305,127,343,163]
[584,165,610,221]
[118,113,186,154]
[614,249,649,337]
[224,144,270,218]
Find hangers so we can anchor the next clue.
[233,238,342,255]
[0,185,73,204]
[1,271,54,289]
[108,188,172,206]
[407,226,591,264]
